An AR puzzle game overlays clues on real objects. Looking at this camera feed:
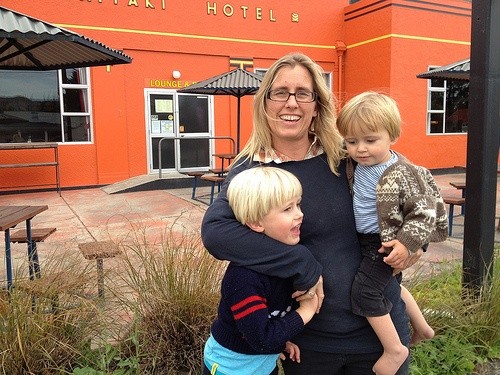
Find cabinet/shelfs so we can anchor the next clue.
[0,142,62,198]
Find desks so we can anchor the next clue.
[0,205,97,320]
[212,153,238,189]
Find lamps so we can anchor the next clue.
[173,71,181,78]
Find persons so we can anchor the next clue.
[337,91,450,375]
[201,53,429,375]
[204,168,318,375]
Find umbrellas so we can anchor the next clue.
[176,66,264,153]
[0,7,133,72]
[416,58,471,80]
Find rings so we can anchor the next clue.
[307,291,314,295]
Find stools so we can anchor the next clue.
[10,227,57,287]
[442,195,465,235]
[20,273,88,326]
[187,168,225,207]
[79,239,122,303]
[449,182,467,214]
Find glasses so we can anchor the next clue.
[267,88,319,103]
